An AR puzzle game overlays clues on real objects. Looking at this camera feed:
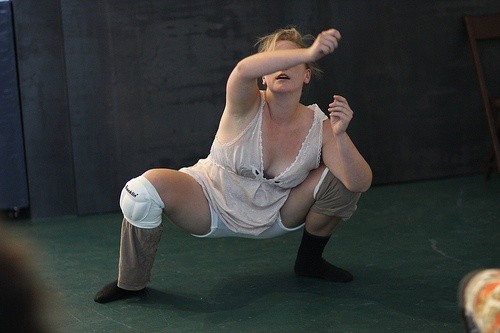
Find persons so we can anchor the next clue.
[94,28,373,303]
